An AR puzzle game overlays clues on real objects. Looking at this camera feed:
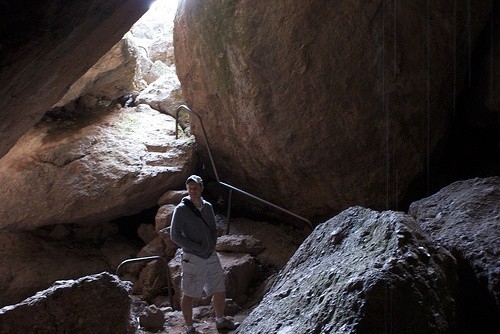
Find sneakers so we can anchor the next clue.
[186,327,202,334]
[215,319,240,331]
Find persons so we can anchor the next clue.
[170,175,240,334]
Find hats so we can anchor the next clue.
[185,175,203,184]
